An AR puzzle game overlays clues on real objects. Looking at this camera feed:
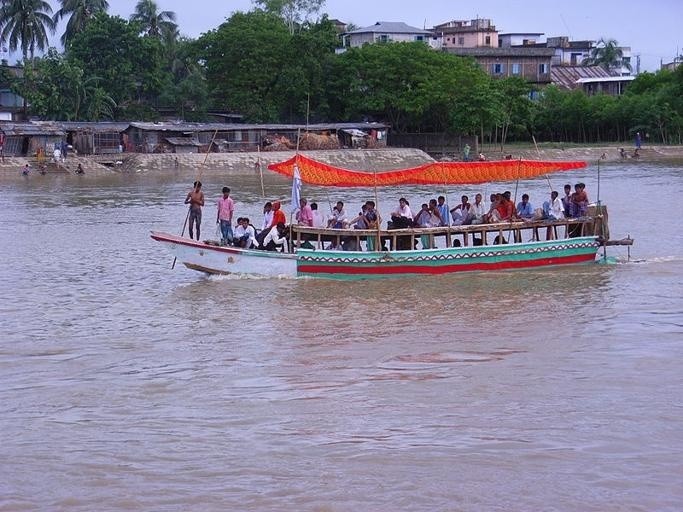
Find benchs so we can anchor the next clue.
[284,215,593,252]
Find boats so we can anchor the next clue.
[149,154,635,278]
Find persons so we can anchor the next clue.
[23,141,85,176]
[635,132,641,148]
[464,144,472,159]
[0,130,7,163]
[477,151,488,162]
